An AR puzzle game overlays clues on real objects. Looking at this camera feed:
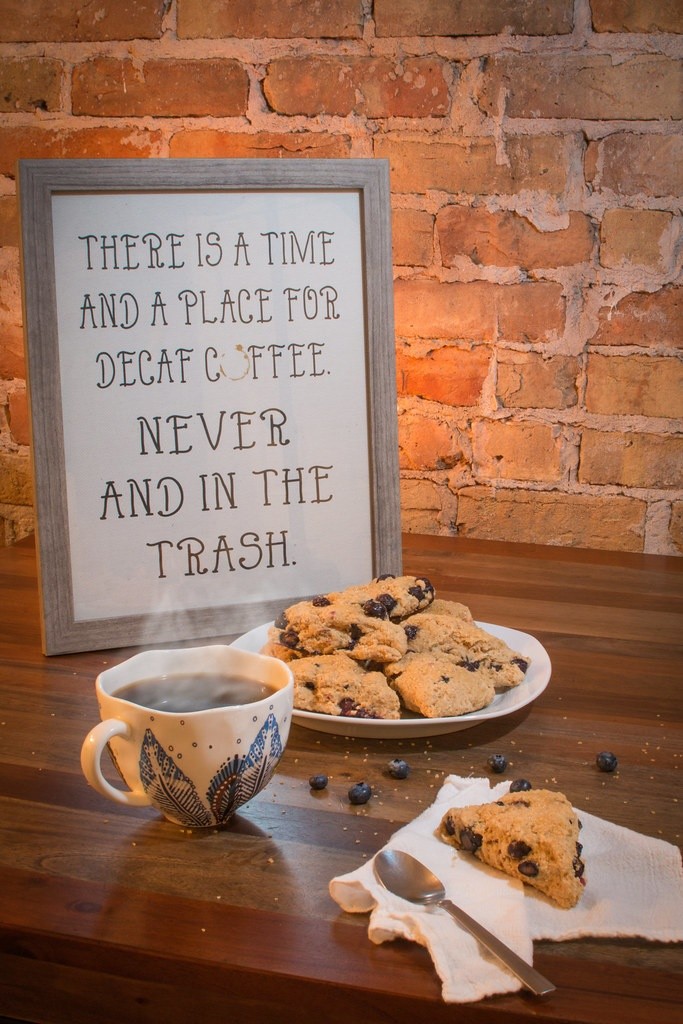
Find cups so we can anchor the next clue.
[81,644,294,827]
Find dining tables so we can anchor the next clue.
[1,532,683,1024]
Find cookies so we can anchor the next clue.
[259,574,532,721]
[428,789,587,911]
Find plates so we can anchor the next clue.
[227,620,553,738]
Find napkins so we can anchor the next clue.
[328,779,683,1002]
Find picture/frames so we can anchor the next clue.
[16,158,401,655]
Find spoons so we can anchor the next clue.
[373,848,557,996]
